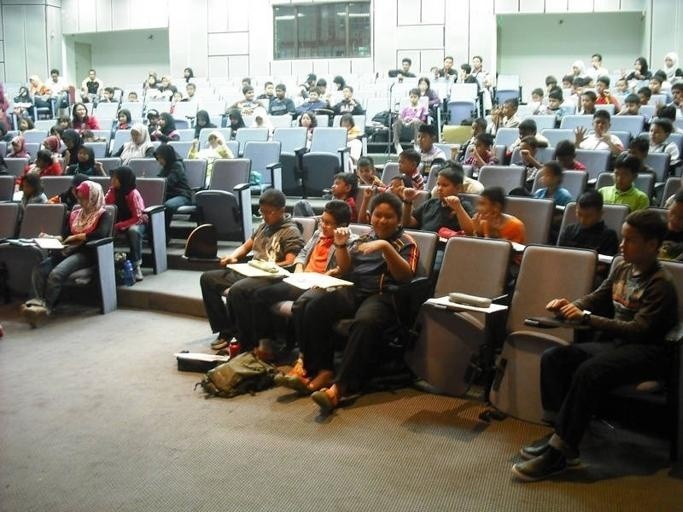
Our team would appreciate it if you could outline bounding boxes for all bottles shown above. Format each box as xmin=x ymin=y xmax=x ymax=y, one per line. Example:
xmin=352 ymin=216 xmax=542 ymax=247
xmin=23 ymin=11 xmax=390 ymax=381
xmin=123 ymin=260 xmax=135 ymax=285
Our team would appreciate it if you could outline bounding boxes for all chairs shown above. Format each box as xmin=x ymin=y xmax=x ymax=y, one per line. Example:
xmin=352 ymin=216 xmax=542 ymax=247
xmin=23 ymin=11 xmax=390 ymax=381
xmin=171 ymin=78 xmax=283 ymax=242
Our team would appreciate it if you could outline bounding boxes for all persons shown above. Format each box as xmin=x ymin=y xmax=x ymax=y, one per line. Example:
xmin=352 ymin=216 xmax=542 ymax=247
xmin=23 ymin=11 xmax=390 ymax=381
xmin=289 ymin=190 xmax=421 ymax=413
xmin=251 ymin=201 xmax=359 ymax=389
xmin=1 ymin=52 xmax=682 ymax=320
xmin=511 ymin=208 xmax=680 ymax=482
xmin=199 ymin=188 xmax=305 ymax=351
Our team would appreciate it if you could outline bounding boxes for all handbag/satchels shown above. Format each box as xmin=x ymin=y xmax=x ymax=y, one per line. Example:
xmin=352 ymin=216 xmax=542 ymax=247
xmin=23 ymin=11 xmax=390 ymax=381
xmin=200 ymin=351 xmax=284 ymax=397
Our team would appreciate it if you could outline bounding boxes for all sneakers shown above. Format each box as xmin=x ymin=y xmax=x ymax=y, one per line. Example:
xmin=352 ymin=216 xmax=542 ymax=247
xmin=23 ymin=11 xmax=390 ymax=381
xmin=511 ymin=454 xmax=566 ymax=481
xmin=211 ymin=331 xmax=230 ymax=349
xmin=20 ymin=304 xmax=48 ymax=328
xmin=521 ymin=440 xmax=580 ymax=465
xmin=134 ymin=266 xmax=144 ymax=280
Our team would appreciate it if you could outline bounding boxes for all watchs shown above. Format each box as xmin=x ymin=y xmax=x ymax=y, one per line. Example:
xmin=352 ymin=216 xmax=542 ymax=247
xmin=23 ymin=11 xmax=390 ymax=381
xmin=583 ymin=309 xmax=591 ymax=320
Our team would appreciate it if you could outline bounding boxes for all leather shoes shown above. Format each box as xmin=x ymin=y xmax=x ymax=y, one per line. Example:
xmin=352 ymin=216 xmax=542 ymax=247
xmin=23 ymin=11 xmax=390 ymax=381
xmin=274 ymin=370 xmax=337 ymax=412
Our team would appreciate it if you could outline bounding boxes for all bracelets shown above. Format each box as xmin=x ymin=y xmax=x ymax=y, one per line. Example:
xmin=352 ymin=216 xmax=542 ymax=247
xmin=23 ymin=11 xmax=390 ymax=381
xmin=334 ymin=243 xmax=347 ymax=249
